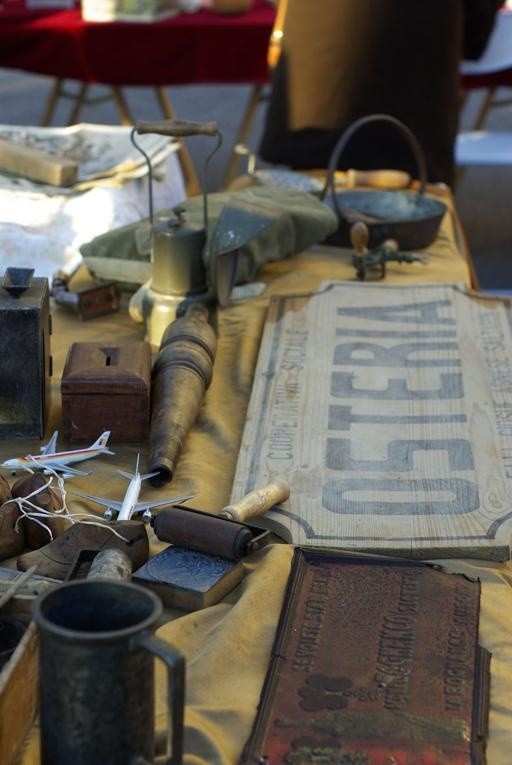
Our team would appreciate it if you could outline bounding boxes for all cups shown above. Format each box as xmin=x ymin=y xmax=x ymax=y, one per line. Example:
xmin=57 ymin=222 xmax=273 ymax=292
xmin=29 ymin=579 xmax=185 ymax=764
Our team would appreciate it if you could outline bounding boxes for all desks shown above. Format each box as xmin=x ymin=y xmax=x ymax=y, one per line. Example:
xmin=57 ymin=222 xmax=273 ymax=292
xmin=0 ymin=169 xmax=511 ymax=765
xmin=0 ymin=0 xmax=276 ymax=199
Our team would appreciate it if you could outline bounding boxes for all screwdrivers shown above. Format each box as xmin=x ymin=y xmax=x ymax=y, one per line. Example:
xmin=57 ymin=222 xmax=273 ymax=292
xmin=324 ymin=169 xmax=410 ymax=189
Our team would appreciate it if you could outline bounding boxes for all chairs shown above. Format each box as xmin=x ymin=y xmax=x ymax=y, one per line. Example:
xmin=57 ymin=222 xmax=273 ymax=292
xmin=220 ymin=2 xmax=502 ymax=195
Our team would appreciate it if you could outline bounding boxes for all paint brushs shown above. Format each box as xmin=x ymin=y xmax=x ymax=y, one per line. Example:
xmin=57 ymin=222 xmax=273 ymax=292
xmin=0 ymin=558 xmax=44 ymax=609
